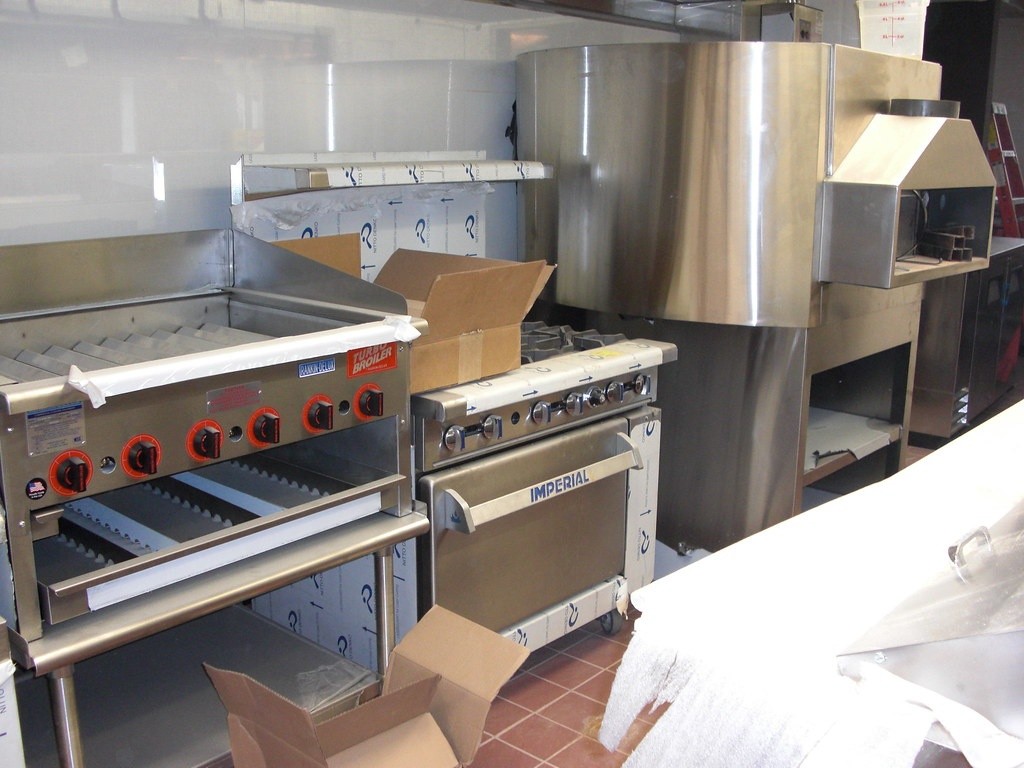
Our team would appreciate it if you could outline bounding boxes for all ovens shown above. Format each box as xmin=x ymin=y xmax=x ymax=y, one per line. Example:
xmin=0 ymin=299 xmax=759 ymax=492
xmin=417 ymin=405 xmax=661 ymax=649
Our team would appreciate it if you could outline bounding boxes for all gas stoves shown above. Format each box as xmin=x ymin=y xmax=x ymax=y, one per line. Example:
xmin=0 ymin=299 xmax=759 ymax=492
xmin=411 ymin=322 xmax=677 ymax=470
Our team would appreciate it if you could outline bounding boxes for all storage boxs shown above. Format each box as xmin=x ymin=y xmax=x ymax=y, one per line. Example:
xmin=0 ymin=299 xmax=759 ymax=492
xmin=269 ymin=233 xmax=560 ymax=395
xmin=202 ymin=601 xmax=532 ymax=768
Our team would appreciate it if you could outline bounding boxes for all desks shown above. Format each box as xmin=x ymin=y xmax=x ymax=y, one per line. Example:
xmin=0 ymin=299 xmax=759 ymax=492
xmin=600 ymin=397 xmax=1023 ymax=768
